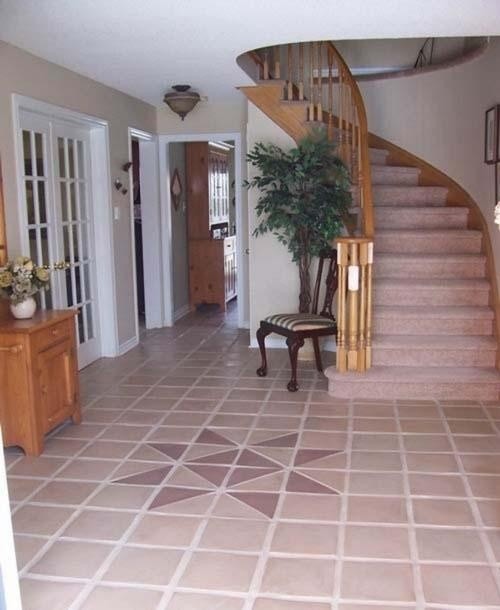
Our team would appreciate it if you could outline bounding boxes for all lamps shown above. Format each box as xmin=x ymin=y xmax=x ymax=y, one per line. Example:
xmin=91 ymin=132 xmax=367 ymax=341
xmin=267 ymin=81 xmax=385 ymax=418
xmin=164 ymin=84 xmax=200 ymax=120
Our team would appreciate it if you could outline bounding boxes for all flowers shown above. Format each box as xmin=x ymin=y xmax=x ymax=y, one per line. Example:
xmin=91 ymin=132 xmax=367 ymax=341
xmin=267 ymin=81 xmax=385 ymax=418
xmin=0 ymin=256 xmax=73 ymax=305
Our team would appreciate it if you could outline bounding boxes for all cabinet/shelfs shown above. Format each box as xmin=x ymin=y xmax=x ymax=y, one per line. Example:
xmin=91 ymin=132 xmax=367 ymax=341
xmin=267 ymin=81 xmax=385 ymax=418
xmin=186 ymin=139 xmax=239 ymax=313
xmin=0 ymin=308 xmax=80 ymax=458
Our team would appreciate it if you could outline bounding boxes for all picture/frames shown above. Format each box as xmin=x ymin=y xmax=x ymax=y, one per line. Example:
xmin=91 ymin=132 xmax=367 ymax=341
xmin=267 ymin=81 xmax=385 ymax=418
xmin=171 ymin=169 xmax=183 ymax=211
xmin=485 ymin=104 xmax=500 ymax=165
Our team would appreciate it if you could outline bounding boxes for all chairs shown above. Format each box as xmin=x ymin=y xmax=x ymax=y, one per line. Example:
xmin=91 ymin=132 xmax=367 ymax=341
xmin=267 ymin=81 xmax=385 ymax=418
xmin=256 ymin=247 xmax=351 ymax=392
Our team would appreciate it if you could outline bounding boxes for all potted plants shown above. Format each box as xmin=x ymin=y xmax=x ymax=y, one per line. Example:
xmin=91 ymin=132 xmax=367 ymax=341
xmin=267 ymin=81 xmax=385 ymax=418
xmin=239 ymin=127 xmax=354 ymax=362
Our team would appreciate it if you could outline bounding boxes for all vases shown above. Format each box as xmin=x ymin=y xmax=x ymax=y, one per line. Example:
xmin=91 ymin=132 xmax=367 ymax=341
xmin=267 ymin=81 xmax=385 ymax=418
xmin=9 ymin=297 xmax=37 ymax=320
